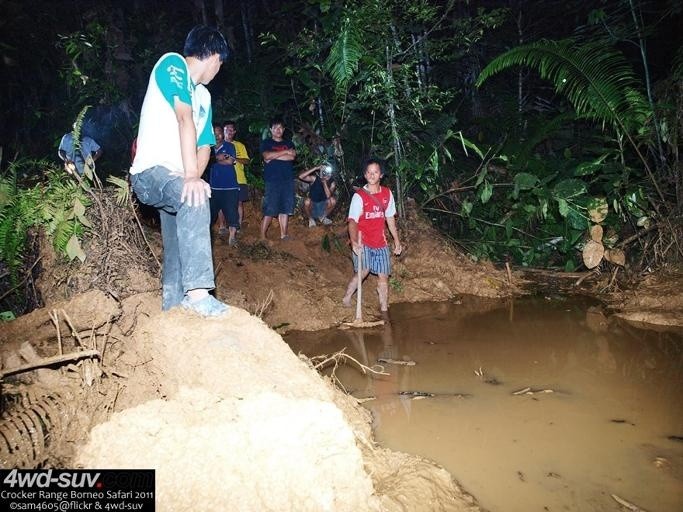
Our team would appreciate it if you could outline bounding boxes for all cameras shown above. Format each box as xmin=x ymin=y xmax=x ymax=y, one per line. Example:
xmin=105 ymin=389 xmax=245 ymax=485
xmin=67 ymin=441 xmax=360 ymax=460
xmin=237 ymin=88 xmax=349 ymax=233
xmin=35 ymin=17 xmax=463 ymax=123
xmin=320 ymin=167 xmax=332 ymax=178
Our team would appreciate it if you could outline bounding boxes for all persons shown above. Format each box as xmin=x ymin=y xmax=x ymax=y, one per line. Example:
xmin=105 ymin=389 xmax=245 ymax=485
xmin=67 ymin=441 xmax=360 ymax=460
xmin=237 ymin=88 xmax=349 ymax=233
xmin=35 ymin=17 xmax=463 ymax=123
xmin=126 ymin=26 xmax=235 ymax=318
xmin=130 ymin=125 xmax=161 ymax=226
xmin=347 ymin=310 xmax=416 ymax=450
xmin=298 ymin=160 xmax=338 ymax=230
xmin=341 ymin=160 xmax=401 ymax=311
xmin=57 ymin=116 xmax=104 ymax=190
xmin=208 ymin=124 xmax=239 ymax=250
xmin=258 ymin=119 xmax=299 ymax=242
xmin=217 ymin=121 xmax=250 ymax=236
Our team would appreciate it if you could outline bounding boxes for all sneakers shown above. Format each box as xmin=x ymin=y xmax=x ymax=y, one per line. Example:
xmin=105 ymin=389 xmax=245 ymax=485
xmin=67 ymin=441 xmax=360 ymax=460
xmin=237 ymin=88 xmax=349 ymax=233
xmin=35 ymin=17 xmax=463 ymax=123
xmin=319 ymin=217 xmax=333 ymax=225
xmin=280 ymin=234 xmax=295 ymax=241
xmin=180 ymin=293 xmax=230 ymax=318
xmin=308 ymin=218 xmax=317 ymax=228
xmin=219 ymin=229 xmax=225 ymax=235
xmin=228 ymin=236 xmax=237 ymax=247
